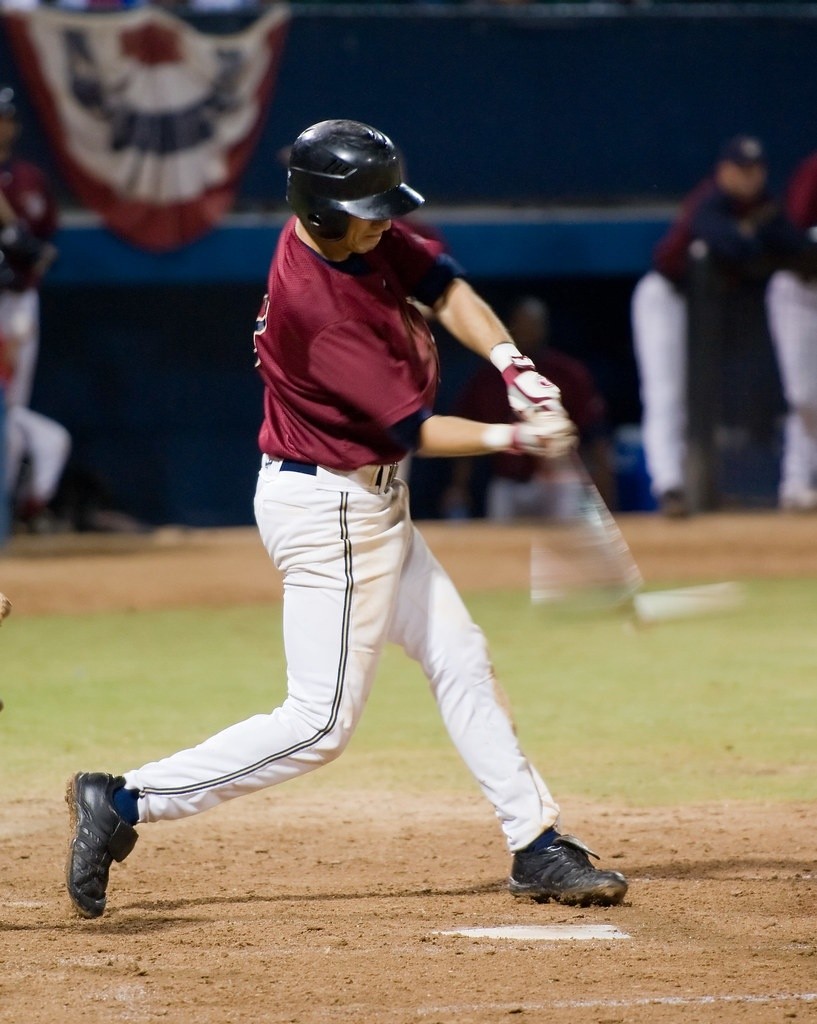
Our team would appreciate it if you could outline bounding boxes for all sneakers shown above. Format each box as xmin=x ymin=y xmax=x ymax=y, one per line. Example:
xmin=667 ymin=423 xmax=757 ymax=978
xmin=505 ymin=832 xmax=628 ymax=903
xmin=61 ymin=770 xmax=139 ymax=918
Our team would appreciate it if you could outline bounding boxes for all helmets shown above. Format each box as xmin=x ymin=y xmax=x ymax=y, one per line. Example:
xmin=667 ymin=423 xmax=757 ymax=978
xmin=284 ymin=119 xmax=426 ymax=243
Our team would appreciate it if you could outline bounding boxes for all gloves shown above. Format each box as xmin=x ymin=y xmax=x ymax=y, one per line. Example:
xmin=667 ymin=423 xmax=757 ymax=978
xmin=509 ymin=416 xmax=580 ymax=459
xmin=502 ymin=354 xmax=570 ymax=421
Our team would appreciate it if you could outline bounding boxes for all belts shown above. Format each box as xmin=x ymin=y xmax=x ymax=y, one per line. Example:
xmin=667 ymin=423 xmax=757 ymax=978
xmin=279 ymin=458 xmax=385 ymax=487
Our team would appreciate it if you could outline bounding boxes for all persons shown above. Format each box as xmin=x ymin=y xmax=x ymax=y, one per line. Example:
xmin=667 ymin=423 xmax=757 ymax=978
xmin=631 ymin=135 xmax=778 ymax=518
xmin=64 ymin=122 xmax=628 ymax=920
xmin=443 ymin=302 xmax=605 ymax=518
xmin=765 ymin=154 xmax=817 ymax=508
xmin=0 ymin=89 xmax=70 ymax=537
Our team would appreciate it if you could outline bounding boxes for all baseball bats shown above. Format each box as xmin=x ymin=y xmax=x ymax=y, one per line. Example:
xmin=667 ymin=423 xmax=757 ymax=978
xmin=532 ymin=448 xmax=646 ymax=613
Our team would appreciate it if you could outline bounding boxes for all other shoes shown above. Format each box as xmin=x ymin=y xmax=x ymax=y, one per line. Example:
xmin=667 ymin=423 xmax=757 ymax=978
xmin=654 ymin=487 xmax=690 ymax=517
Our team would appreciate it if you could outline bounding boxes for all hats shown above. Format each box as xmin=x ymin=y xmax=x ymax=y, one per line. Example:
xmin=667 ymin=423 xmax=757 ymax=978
xmin=720 ymin=133 xmax=780 ymax=171
